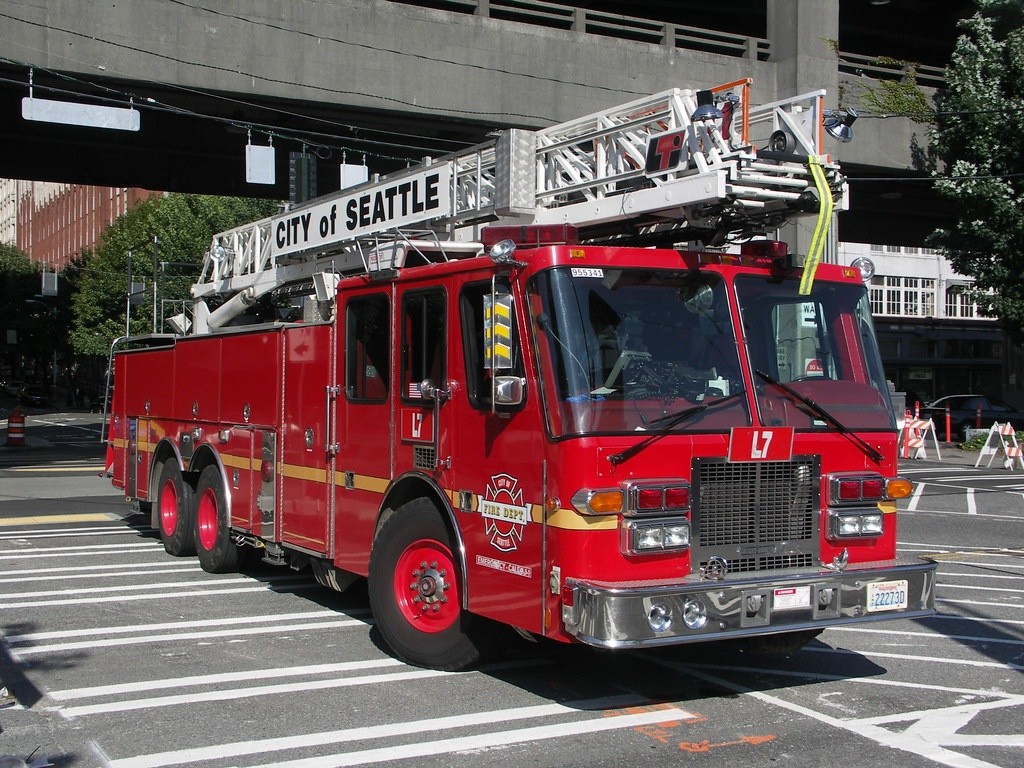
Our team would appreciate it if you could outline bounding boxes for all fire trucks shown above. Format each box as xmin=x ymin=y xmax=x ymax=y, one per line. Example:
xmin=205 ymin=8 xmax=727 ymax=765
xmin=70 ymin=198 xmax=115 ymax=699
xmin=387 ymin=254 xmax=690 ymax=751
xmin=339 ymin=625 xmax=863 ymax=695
xmin=101 ymin=79 xmax=937 ymax=672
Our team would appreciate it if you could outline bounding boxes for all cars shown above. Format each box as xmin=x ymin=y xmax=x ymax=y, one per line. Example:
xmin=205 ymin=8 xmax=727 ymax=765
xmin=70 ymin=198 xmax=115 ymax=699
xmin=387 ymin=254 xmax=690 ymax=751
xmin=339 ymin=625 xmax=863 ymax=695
xmin=90 ymin=395 xmax=112 ymax=414
xmin=911 ymin=395 xmax=1024 ymax=439
xmin=0 ymin=378 xmax=24 ymax=396
xmin=21 ymin=384 xmax=46 ymax=407
